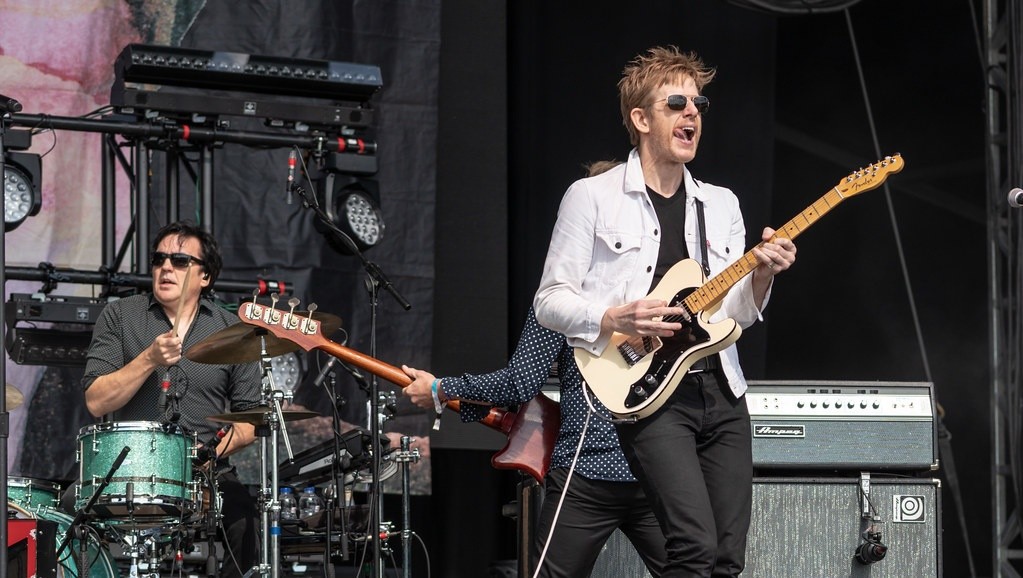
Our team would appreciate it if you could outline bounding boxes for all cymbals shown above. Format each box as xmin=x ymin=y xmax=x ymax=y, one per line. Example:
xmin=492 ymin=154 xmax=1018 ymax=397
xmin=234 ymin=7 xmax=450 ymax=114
xmin=5 ymin=382 xmax=24 ymax=411
xmin=181 ymin=308 xmax=343 ymax=365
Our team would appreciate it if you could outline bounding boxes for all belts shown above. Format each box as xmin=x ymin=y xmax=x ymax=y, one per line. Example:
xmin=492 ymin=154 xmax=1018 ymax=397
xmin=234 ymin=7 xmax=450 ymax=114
xmin=687 ymin=355 xmax=721 ymax=373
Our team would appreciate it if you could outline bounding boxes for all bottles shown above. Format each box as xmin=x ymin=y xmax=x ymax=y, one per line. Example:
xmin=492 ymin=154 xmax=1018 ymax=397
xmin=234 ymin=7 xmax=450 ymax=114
xmin=277 ymin=487 xmax=297 ymax=521
xmin=346 ymin=488 xmax=358 ymax=507
xmin=299 ymin=487 xmax=324 ymax=519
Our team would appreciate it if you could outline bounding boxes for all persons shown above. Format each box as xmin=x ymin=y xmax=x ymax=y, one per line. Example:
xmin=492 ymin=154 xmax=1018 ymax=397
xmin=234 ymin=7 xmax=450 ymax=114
xmin=529 ymin=48 xmax=799 ymax=577
xmin=82 ymin=222 xmax=265 ymax=578
xmin=401 ymin=160 xmax=673 ymax=575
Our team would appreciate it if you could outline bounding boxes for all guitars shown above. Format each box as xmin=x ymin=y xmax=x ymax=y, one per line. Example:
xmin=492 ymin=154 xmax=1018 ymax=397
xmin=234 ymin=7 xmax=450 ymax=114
xmin=572 ymin=152 xmax=905 ymax=419
xmin=238 ymin=288 xmax=561 ymax=484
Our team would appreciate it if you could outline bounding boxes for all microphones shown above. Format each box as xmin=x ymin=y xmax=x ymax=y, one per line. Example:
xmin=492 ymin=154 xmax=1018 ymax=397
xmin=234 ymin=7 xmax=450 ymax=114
xmin=856 ymin=542 xmax=887 ymax=565
xmin=313 ymin=340 xmax=347 ymax=388
xmin=192 ymin=425 xmax=232 ymax=467
xmin=158 ymin=371 xmax=170 ymax=412
xmin=286 ymin=149 xmax=297 ymax=204
xmin=1007 ymin=187 xmax=1023 ymax=208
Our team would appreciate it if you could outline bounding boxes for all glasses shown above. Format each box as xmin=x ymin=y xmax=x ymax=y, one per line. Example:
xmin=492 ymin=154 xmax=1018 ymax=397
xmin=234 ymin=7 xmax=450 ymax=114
xmin=149 ymin=251 xmax=205 ymax=268
xmin=643 ymin=95 xmax=709 ymax=113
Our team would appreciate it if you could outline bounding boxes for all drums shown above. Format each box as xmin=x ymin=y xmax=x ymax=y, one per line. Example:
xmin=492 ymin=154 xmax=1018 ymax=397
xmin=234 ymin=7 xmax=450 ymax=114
xmin=7 ymin=475 xmax=62 ymax=510
xmin=71 ymin=420 xmax=202 ymax=520
xmin=7 ymin=501 xmax=118 ymax=578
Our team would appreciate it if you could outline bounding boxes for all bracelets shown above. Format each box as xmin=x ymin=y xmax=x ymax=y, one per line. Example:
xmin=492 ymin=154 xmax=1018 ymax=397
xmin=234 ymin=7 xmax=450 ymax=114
xmin=432 ymin=377 xmax=451 ymax=431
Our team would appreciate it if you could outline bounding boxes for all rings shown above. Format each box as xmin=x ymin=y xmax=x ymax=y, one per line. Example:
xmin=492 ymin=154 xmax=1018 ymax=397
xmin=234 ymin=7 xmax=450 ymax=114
xmin=768 ymin=262 xmax=776 ymax=268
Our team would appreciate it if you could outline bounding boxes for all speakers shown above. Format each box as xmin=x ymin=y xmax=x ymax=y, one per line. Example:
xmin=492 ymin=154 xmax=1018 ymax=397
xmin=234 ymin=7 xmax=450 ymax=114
xmin=589 ymin=475 xmax=943 ymax=578
